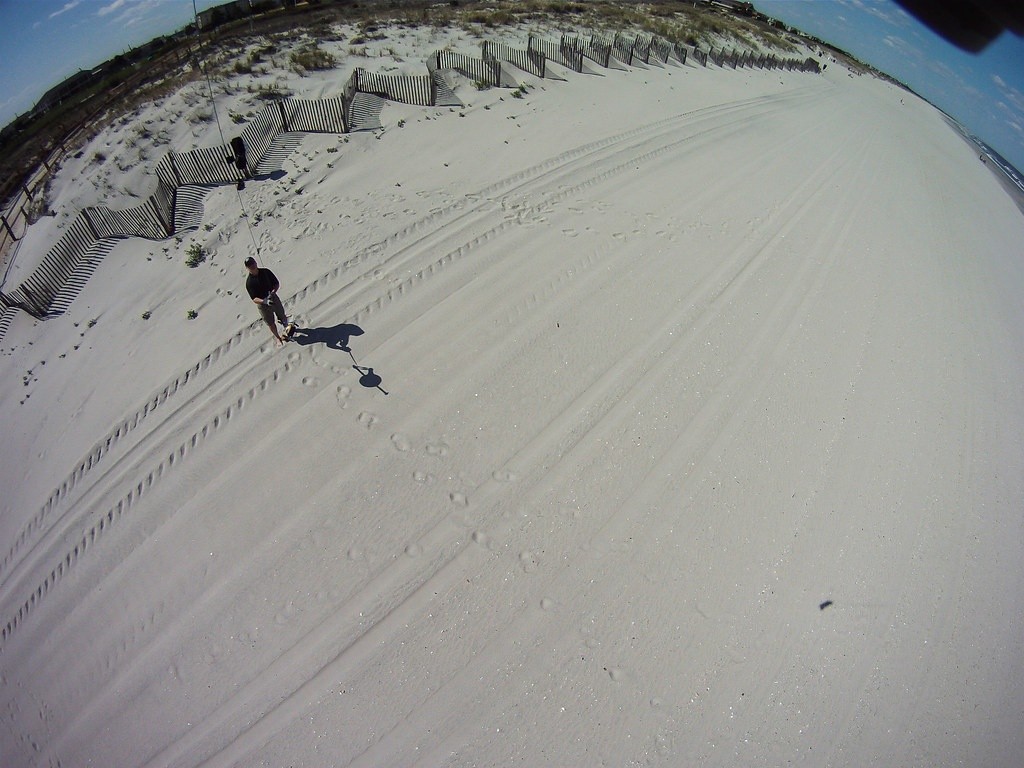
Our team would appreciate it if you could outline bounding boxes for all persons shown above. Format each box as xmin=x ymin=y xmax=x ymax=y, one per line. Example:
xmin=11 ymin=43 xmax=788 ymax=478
xmin=244 ymin=257 xmax=287 ymax=346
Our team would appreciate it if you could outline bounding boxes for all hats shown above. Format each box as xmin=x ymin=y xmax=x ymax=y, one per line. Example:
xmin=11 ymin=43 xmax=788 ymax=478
xmin=244 ymin=257 xmax=255 ymax=267
